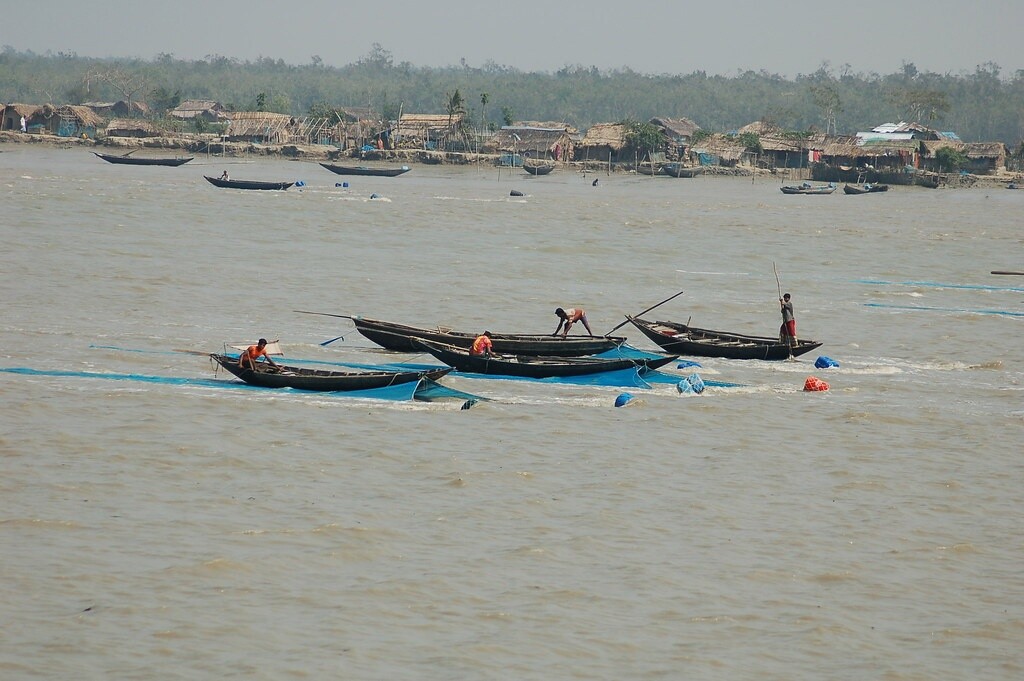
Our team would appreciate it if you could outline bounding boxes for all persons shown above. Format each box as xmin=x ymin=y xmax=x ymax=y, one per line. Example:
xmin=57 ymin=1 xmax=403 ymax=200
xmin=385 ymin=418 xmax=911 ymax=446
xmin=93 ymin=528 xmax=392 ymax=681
xmin=593 ymin=179 xmax=599 ymax=186
xmin=378 ymin=134 xmax=406 ymax=150
xmin=555 ymin=145 xmax=568 ymax=162
xmin=165 ymin=107 xmax=169 ymax=116
xmin=469 ymin=331 xmax=496 ymax=357
xmin=20 ymin=115 xmax=26 ymax=133
xmin=81 ymin=133 xmax=86 ymax=145
xmin=666 ymin=147 xmax=679 ymax=161
xmin=238 ymin=339 xmax=284 ymax=372
xmin=780 ymin=293 xmax=798 ymax=345
xmin=220 ymin=170 xmax=229 ymax=181
xmin=552 ymin=308 xmax=592 ymax=338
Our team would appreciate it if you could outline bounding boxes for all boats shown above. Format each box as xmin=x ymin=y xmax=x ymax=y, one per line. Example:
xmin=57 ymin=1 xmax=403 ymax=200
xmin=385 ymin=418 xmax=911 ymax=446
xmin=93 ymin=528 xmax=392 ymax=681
xmin=842 ymin=172 xmax=889 ymax=194
xmin=318 ymin=163 xmax=412 ymax=177
xmin=522 ymin=164 xmax=556 ymax=175
xmin=356 ymin=325 xmax=680 ymax=378
xmin=204 ymin=175 xmax=294 ymax=191
xmin=420 ymin=151 xmax=444 ymax=165
xmin=92 ymin=149 xmax=194 ymax=167
xmin=637 ymin=160 xmax=704 ymax=178
xmin=293 ymin=311 xmax=628 ymax=352
xmin=172 ymin=342 xmax=458 ymax=392
xmin=625 ymin=314 xmax=824 ymax=362
xmin=911 ymin=164 xmax=942 ymax=189
xmin=779 ymin=181 xmax=838 ymax=194
